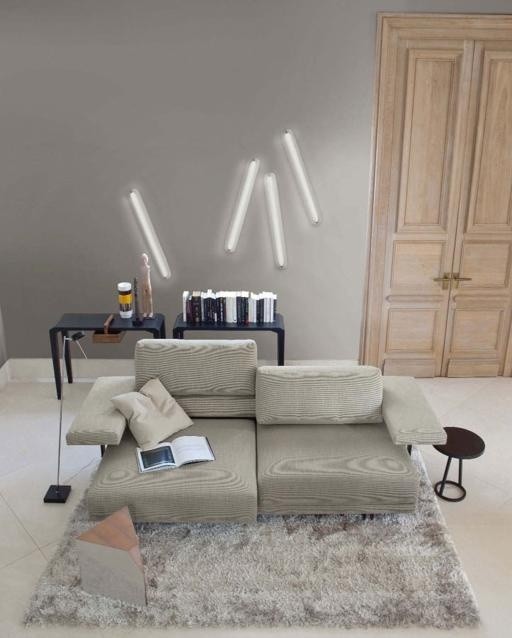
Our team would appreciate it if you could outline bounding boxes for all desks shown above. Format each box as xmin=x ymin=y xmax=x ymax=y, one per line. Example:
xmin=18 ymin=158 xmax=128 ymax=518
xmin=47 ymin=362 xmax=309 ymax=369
xmin=49 ymin=313 xmax=285 ymax=400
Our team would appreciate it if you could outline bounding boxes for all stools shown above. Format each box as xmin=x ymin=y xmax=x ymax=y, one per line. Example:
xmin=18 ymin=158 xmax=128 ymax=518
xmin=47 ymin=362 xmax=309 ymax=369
xmin=433 ymin=426 xmax=485 ymax=503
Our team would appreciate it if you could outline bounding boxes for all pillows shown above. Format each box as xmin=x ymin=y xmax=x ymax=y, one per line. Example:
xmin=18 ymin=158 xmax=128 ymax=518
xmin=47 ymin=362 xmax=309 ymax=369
xmin=111 ymin=377 xmax=194 ymax=453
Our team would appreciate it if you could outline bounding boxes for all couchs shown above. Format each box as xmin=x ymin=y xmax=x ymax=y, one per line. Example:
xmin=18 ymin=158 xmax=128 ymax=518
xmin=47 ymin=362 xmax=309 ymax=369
xmin=64 ymin=338 xmax=448 ymax=527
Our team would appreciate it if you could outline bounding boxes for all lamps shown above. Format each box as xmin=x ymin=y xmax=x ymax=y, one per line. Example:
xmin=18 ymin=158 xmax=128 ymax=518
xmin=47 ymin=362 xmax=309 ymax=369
xmin=43 ymin=331 xmax=86 ymax=503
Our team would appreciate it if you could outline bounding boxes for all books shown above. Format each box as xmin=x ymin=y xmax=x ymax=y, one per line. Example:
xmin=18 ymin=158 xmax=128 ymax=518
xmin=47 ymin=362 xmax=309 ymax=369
xmin=134 ymin=433 xmax=215 ymax=474
xmin=182 ymin=289 xmax=278 ymax=325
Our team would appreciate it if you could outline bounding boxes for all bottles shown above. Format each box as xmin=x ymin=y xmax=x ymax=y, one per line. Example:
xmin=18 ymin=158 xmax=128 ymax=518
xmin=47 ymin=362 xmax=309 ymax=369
xmin=117 ymin=281 xmax=133 ymax=319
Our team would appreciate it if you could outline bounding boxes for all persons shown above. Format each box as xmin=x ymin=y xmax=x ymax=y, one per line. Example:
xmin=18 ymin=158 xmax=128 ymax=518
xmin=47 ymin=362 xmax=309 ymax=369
xmin=139 ymin=253 xmax=153 ymax=318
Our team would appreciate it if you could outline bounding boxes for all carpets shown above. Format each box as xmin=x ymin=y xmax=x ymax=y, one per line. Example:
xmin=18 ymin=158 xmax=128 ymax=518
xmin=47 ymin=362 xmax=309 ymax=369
xmin=20 ymin=444 xmax=483 ymax=630
